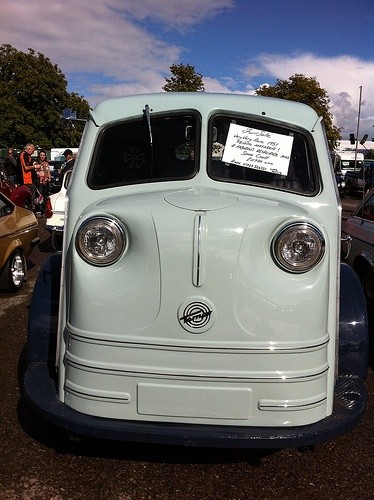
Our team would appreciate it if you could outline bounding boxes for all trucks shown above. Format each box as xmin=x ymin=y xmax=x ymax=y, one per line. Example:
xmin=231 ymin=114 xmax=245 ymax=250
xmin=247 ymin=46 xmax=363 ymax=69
xmin=333 ymin=151 xmax=365 ymax=187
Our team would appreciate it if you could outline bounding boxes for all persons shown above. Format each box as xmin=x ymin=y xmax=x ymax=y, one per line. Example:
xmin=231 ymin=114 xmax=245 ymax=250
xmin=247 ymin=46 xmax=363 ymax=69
xmin=59 ymin=149 xmax=75 ymax=187
xmin=16 ymin=144 xmax=41 ymax=206
xmin=33 ymin=151 xmax=51 ymax=215
xmin=3 ymin=148 xmax=17 ymax=183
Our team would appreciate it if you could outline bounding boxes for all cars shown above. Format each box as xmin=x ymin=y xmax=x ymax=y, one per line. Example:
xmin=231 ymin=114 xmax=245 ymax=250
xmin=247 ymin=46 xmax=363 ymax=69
xmin=15 ymin=91 xmax=371 ymax=455
xmin=0 ymin=143 xmax=79 ymax=214
xmin=44 ymin=169 xmax=73 ymax=232
xmin=341 ymin=187 xmax=374 ymax=305
xmin=0 ymin=190 xmax=41 ymax=294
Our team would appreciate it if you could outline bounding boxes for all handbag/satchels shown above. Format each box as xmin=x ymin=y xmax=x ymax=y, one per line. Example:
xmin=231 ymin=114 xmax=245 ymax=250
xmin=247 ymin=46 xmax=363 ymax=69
xmin=33 ymin=188 xmax=44 ymax=205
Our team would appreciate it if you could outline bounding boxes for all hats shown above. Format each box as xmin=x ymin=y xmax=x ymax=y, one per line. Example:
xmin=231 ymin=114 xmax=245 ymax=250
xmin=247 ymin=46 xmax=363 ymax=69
xmin=62 ymin=149 xmax=72 ymax=155
